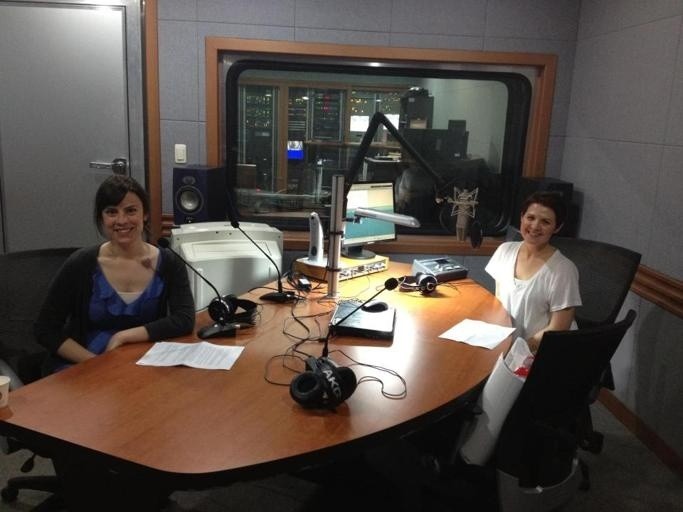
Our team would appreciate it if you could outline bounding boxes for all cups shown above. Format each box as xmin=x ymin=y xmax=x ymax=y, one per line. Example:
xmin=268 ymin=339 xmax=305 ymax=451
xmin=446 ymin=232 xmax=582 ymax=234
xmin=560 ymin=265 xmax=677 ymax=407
xmin=0 ymin=375 xmax=11 ymax=409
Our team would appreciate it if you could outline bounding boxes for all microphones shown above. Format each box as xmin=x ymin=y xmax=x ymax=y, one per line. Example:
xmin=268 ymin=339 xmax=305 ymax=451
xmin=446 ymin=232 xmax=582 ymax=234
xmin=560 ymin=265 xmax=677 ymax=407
xmin=452 ymin=187 xmax=477 ymax=241
xmin=322 ymin=278 xmax=398 ymax=357
xmin=157 ymin=237 xmax=236 ymax=339
xmin=231 ymin=219 xmax=295 ymax=302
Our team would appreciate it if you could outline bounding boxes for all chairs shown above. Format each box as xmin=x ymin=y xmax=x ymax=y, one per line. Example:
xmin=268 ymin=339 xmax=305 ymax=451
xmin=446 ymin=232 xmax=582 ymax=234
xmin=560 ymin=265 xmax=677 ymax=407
xmin=475 ymin=236 xmax=640 ymax=466
xmin=0 ymin=240 xmax=98 ymax=509
xmin=420 ymin=303 xmax=636 ymax=507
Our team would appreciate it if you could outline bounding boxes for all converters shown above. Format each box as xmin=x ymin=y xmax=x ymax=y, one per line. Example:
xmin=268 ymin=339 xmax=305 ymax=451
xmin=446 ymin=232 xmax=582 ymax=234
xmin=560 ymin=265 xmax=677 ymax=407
xmin=298 ymin=278 xmax=312 ymax=289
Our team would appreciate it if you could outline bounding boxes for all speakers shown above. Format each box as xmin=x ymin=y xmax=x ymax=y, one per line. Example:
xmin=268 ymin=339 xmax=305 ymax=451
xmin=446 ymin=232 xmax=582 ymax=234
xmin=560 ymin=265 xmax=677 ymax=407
xmin=172 ymin=164 xmax=229 ymax=224
xmin=308 ymin=211 xmax=324 ymax=261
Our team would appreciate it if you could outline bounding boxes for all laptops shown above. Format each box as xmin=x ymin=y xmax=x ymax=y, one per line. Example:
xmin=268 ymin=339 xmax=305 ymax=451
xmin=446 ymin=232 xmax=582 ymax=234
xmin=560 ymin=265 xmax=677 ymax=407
xmin=329 ymin=298 xmax=395 ymax=341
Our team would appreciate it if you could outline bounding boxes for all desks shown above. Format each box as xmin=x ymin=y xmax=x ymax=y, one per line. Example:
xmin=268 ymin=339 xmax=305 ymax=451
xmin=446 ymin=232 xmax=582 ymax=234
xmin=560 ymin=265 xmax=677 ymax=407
xmin=1 ymin=255 xmax=515 ymax=502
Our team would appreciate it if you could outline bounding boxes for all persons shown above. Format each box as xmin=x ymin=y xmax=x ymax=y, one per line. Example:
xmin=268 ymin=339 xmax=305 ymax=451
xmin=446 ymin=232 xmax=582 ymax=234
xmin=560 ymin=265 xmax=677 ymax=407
xmin=484 ymin=190 xmax=584 ymax=365
xmin=35 ymin=174 xmax=195 ymax=374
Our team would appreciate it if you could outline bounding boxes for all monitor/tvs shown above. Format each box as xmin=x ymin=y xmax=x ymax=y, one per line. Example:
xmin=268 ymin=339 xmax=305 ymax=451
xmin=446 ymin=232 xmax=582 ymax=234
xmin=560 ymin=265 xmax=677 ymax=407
xmin=287 ymin=140 xmax=304 ymax=161
xmin=341 ymin=180 xmax=397 ymax=259
xmin=383 ymin=113 xmax=400 ymax=130
xmin=350 ymin=114 xmax=369 ymax=132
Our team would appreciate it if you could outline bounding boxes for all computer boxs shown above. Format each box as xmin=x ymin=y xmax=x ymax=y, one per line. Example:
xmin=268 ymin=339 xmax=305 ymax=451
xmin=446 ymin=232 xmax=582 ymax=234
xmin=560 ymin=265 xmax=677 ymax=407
xmin=295 ymin=250 xmax=389 ymax=284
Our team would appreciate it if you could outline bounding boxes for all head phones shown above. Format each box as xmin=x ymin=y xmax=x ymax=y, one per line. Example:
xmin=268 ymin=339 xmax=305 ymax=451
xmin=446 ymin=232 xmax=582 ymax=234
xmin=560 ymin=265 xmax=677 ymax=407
xmin=397 ymin=272 xmax=437 ymax=294
xmin=291 ymin=356 xmax=356 ymax=408
xmin=208 ymin=294 xmax=256 ymax=324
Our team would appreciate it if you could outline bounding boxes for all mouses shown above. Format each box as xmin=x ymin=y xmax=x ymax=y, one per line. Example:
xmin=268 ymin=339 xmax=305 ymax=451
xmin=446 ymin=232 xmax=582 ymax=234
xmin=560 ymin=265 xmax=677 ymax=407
xmin=363 ymin=300 xmax=388 ymax=312
